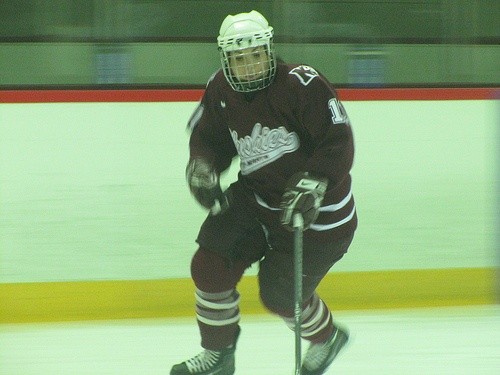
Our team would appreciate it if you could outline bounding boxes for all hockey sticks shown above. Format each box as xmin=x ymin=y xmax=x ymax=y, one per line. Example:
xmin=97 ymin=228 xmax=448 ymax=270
xmin=292 ymin=212 xmax=304 ymax=375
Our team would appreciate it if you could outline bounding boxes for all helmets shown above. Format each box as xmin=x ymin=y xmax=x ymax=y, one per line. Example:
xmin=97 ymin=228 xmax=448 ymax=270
xmin=217 ymin=10 xmax=277 ymax=92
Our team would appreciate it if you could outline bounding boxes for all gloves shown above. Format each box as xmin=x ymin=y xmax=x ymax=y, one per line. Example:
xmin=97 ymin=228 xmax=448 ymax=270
xmin=185 ymin=160 xmax=225 ymax=210
xmin=279 ymin=172 xmax=329 ymax=232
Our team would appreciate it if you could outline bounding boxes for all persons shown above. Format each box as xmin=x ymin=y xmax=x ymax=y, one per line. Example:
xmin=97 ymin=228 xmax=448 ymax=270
xmin=171 ymin=10 xmax=359 ymax=375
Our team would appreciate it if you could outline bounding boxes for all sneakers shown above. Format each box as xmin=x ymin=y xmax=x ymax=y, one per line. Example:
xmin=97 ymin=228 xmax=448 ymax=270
xmin=301 ymin=326 xmax=348 ymax=375
xmin=170 ymin=348 xmax=235 ymax=375
